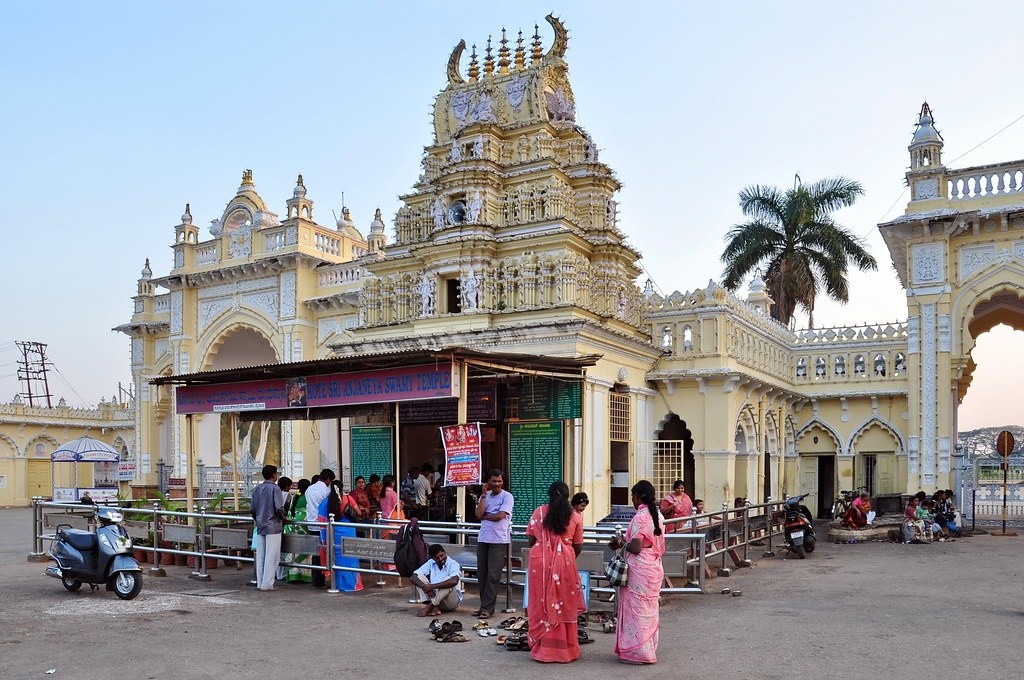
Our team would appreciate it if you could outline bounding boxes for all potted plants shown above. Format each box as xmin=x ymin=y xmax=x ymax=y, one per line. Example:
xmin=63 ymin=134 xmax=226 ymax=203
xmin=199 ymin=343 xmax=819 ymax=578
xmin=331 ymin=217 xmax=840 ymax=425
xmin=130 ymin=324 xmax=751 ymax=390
xmin=114 ymin=491 xmax=249 ymax=569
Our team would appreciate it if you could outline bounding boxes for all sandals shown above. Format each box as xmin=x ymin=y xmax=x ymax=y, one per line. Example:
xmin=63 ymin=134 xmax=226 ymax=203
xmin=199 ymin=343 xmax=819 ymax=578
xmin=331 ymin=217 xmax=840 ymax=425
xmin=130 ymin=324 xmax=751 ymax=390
xmin=472 ymin=610 xmax=482 ymax=616
xmin=478 ymin=611 xmax=491 ymax=619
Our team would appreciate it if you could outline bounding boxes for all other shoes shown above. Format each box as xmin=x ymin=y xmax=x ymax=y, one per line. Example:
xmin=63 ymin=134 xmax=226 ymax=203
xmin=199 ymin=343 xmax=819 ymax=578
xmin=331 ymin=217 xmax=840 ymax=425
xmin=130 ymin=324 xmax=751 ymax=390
xmin=832 ymin=529 xmax=974 ymax=545
xmin=262 ymin=587 xmax=279 ymax=592
xmin=608 ymin=619 xmax=616 ymax=631
xmin=472 ymin=623 xmax=485 ymax=630
xmin=603 ymin=622 xmax=610 ymax=632
xmin=312 ymin=584 xmax=330 ymax=589
xmin=487 ymin=628 xmax=497 ymax=636
xmin=619 ymin=659 xmax=645 ymax=665
xmin=291 ymin=580 xmax=300 ymax=584
xmin=428 ymin=618 xmax=470 ymax=642
xmin=478 ymin=619 xmax=489 ymax=628
xmin=278 ymin=577 xmax=286 ymax=581
xmin=477 ymin=629 xmax=488 ymax=637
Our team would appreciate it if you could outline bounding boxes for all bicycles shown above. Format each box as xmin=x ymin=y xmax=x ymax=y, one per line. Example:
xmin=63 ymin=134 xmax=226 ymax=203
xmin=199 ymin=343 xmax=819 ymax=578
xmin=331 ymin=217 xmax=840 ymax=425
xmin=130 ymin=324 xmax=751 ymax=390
xmin=831 ymin=485 xmax=869 ymax=529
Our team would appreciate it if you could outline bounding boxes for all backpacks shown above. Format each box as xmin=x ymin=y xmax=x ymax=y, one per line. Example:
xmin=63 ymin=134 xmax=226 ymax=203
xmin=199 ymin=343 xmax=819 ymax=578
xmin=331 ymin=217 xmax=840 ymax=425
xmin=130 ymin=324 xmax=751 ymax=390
xmin=367 ymin=485 xmax=382 ymax=514
xmin=394 ymin=523 xmax=420 ymax=577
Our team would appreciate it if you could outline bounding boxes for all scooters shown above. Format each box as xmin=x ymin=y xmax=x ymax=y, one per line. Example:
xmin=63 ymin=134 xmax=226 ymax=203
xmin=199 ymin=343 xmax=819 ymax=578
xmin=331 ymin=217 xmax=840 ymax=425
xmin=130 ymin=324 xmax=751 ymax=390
xmin=778 ymin=492 xmax=816 ymax=559
xmin=45 ymin=495 xmax=145 ymax=601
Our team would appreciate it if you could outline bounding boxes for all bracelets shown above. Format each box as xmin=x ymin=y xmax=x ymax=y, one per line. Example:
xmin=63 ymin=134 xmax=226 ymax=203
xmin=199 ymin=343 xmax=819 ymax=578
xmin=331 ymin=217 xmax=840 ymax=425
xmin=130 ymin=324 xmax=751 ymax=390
xmin=481 ymin=495 xmax=486 ymax=499
xmin=617 ymin=538 xmax=626 ymax=547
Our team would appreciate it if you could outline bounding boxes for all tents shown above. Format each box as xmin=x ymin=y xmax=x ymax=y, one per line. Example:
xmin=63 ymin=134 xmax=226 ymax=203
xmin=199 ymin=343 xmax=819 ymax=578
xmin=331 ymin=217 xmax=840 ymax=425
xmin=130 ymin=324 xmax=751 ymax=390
xmin=51 ymin=434 xmax=120 ymax=489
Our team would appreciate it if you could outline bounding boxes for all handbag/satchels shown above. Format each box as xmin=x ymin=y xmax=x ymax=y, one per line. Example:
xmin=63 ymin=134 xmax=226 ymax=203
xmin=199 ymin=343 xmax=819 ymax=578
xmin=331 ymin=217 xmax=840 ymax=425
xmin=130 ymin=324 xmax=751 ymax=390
xmin=945 ymin=506 xmax=954 ymax=521
xmin=951 ymin=508 xmax=961 ymax=527
xmin=604 ymin=543 xmax=629 ymax=587
xmin=866 ymin=510 xmax=876 ymax=524
xmin=344 ymin=495 xmax=359 ymax=523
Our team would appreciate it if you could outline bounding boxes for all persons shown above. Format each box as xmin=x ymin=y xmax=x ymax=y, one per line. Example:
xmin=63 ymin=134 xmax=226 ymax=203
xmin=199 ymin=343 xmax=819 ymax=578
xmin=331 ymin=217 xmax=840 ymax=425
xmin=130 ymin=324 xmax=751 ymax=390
xmin=250 ymin=465 xmax=290 ymax=591
xmin=410 ymin=544 xmax=464 ymax=617
xmin=276 ymin=463 xmax=488 ymax=589
xmin=846 ymin=493 xmax=872 ymax=529
xmin=905 ymin=489 xmax=961 ymax=544
xmin=607 ymin=480 xmax=665 ymax=664
xmin=526 ymin=481 xmax=589 ymax=662
xmin=660 ymin=480 xmax=723 ymax=552
xmin=471 ymin=469 xmax=514 ymax=619
xmin=420 ymin=277 xmax=430 ymax=316
xmin=461 ymin=276 xmax=482 ymax=308
xmin=159 ymin=517 xmax=177 ymax=530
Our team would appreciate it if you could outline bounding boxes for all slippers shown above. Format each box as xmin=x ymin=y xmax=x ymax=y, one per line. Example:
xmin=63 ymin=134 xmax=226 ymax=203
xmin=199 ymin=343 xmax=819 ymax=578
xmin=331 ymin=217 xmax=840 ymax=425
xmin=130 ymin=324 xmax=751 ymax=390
xmin=496 ymin=616 xmax=595 ymax=651
